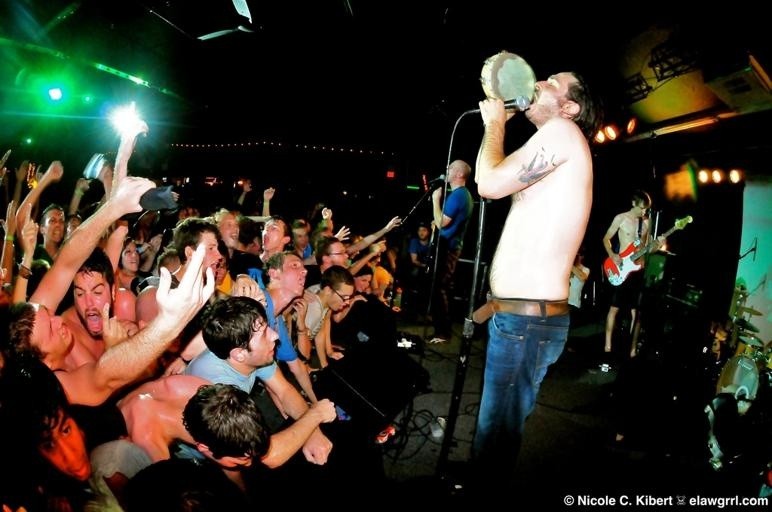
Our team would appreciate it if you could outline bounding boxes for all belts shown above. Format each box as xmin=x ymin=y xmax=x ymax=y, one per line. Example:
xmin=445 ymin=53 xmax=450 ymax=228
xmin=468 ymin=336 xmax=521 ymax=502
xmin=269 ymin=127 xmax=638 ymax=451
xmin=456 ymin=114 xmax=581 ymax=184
xmin=472 ymin=297 xmax=568 ymax=324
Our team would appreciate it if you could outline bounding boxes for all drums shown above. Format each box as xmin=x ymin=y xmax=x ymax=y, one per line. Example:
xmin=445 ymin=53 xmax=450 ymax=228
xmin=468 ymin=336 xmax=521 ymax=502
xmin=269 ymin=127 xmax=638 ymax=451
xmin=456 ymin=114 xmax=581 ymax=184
xmin=764 ymin=346 xmax=772 ymax=370
xmin=480 ymin=50 xmax=536 ymax=113
xmin=716 ymin=352 xmax=772 ymax=417
xmin=604 ymin=215 xmax=693 ymax=287
xmin=733 ymin=335 xmax=764 ymax=356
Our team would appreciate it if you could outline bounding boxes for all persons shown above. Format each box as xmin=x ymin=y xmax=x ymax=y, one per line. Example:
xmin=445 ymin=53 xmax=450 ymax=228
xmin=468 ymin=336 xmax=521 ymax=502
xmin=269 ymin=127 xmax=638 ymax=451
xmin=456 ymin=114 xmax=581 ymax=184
xmin=603 ymin=190 xmax=668 ymax=359
xmin=1 ymin=92 xmax=476 ymax=512
xmin=568 ymin=247 xmax=592 ymax=326
xmin=474 ymin=70 xmax=595 ymax=470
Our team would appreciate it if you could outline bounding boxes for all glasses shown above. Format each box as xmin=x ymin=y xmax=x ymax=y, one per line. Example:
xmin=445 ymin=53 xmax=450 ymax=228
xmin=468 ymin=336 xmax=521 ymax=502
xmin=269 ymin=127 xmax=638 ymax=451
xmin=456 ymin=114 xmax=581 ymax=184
xmin=330 ymin=251 xmax=348 ymax=256
xmin=332 ymin=288 xmax=353 ymax=302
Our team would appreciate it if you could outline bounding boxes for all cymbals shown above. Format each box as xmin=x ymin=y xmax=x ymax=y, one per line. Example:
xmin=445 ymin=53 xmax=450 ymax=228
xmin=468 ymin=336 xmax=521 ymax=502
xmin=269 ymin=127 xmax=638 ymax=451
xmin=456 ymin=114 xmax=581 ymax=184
xmin=735 ymin=318 xmax=760 ymax=333
xmin=741 ymin=306 xmax=762 ymax=317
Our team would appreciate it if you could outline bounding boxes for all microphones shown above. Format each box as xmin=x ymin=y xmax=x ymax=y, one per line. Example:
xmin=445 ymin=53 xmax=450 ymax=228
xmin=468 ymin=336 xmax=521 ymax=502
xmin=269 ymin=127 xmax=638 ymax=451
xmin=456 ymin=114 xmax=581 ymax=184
xmin=472 ymin=95 xmax=530 ymax=113
xmin=426 ymin=174 xmax=446 ymax=185
xmin=753 ymin=240 xmax=757 ymax=260
